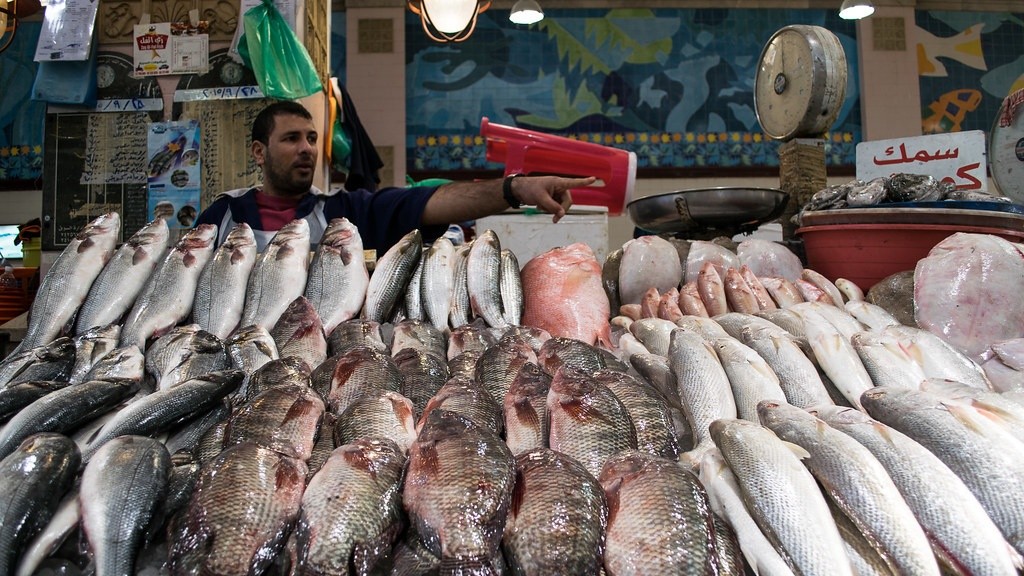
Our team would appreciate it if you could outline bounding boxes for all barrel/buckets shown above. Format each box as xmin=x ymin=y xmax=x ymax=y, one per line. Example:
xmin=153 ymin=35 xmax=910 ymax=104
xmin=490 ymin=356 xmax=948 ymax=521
xmin=21 ymin=238 xmax=40 ymax=267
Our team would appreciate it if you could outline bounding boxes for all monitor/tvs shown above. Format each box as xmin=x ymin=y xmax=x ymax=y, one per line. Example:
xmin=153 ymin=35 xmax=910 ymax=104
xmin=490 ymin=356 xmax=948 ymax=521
xmin=0 ymin=224 xmax=23 ymax=259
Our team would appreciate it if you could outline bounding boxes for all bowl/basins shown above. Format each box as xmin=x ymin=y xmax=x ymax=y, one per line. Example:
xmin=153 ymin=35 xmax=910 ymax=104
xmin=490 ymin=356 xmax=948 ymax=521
xmin=796 ymin=202 xmax=1024 ymax=296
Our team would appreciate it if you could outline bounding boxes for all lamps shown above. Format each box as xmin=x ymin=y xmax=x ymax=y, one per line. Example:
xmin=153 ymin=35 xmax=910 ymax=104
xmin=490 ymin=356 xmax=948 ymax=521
xmin=838 ymin=0 xmax=875 ymax=20
xmin=0 ymin=0 xmax=17 ymax=51
xmin=407 ymin=0 xmax=492 ymax=41
xmin=509 ymin=0 xmax=544 ymax=25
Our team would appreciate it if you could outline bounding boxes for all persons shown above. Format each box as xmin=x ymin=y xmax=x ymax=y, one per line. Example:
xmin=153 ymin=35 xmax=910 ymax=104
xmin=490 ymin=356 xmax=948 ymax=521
xmin=194 ymin=100 xmax=596 ymax=253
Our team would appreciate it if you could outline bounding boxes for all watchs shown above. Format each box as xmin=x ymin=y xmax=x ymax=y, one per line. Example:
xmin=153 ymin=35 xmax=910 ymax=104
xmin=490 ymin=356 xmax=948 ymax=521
xmin=504 ymin=173 xmax=525 ymax=209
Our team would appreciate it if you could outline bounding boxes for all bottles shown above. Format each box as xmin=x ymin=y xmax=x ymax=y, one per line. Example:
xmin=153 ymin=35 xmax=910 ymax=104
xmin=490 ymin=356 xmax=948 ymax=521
xmin=0 ymin=265 xmax=18 ymax=288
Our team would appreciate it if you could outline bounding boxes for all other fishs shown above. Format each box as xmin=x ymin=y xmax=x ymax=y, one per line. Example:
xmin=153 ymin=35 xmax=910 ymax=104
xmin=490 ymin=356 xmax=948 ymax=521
xmin=0 ymin=203 xmax=1019 ymax=576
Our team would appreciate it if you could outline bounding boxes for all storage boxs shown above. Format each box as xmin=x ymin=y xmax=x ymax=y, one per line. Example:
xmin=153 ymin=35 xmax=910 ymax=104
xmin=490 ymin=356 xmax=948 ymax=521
xmin=477 ymin=206 xmax=610 ymax=273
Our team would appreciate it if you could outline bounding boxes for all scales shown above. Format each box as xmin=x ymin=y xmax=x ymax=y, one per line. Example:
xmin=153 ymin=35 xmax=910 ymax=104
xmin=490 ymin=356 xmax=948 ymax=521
xmin=624 ymin=24 xmax=849 ymax=272
xmin=986 ymin=87 xmax=1024 ymax=205
